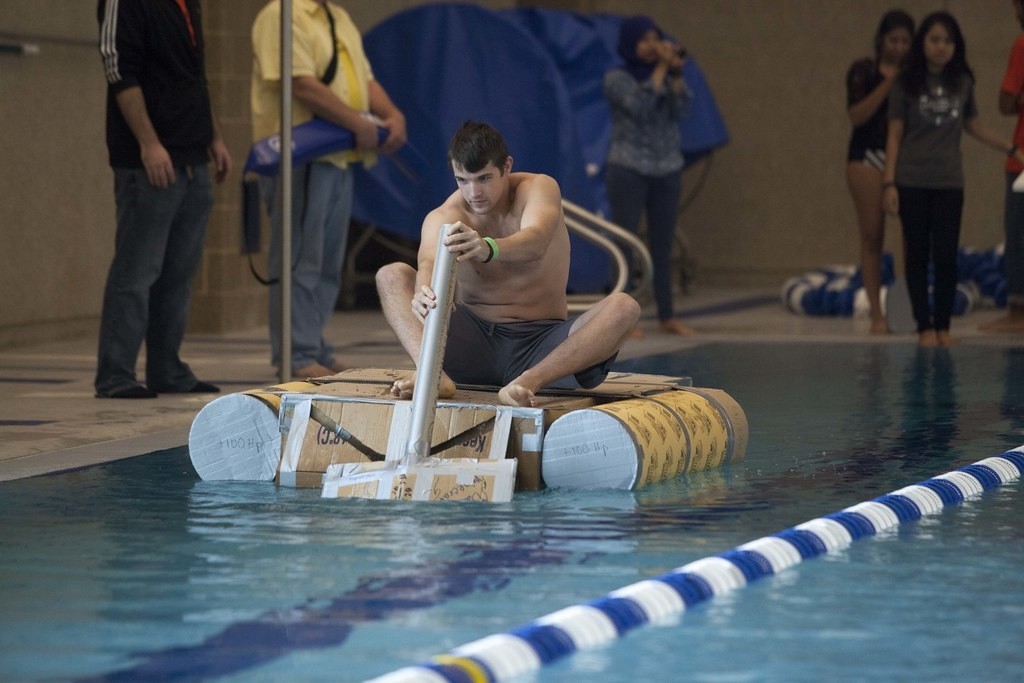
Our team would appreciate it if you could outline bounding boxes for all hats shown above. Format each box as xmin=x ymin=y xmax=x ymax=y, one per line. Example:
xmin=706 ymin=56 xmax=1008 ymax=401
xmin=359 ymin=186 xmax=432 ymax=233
xmin=617 ymin=16 xmax=663 ymax=56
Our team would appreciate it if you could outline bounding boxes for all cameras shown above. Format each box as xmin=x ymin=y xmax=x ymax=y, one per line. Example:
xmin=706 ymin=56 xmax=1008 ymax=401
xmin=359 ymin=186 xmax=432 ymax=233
xmin=671 ymin=44 xmax=688 ymax=58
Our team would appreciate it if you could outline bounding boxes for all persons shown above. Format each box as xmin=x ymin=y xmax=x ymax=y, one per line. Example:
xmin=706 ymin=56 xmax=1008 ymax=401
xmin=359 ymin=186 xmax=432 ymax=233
xmin=977 ymin=0 xmax=1024 ymax=330
xmin=95 ymin=0 xmax=231 ymax=400
xmin=243 ymin=0 xmax=407 ymax=378
xmin=884 ymin=11 xmax=1024 ymax=346
xmin=844 ymin=9 xmax=916 ymax=335
xmin=602 ymin=14 xmax=696 ymax=340
xmin=375 ymin=119 xmax=641 ymax=407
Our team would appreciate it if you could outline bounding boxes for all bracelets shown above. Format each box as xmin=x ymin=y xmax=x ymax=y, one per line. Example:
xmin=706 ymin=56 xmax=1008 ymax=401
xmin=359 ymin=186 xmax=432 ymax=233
xmin=482 ymin=237 xmax=499 ymax=264
xmin=1009 ymin=144 xmax=1017 ymax=155
xmin=885 ymin=181 xmax=895 ymax=187
